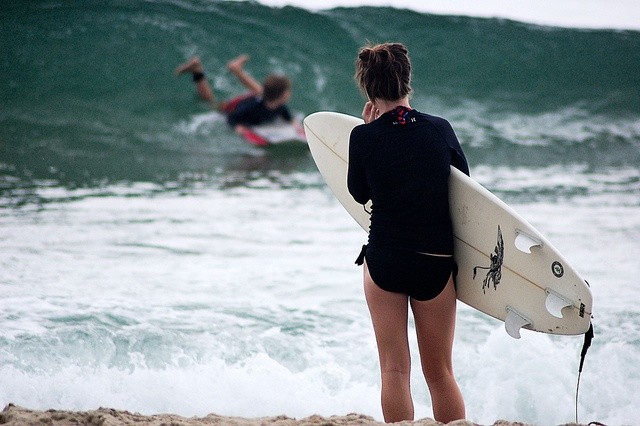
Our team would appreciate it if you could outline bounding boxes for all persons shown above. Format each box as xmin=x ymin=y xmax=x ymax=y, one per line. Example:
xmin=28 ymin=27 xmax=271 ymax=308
xmin=176 ymin=53 xmax=300 ymax=136
xmin=346 ymin=37 xmax=470 ymax=423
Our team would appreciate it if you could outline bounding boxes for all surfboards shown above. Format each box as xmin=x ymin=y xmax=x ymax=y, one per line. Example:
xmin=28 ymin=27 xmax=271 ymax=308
xmin=303 ymin=112 xmax=592 ymax=339
